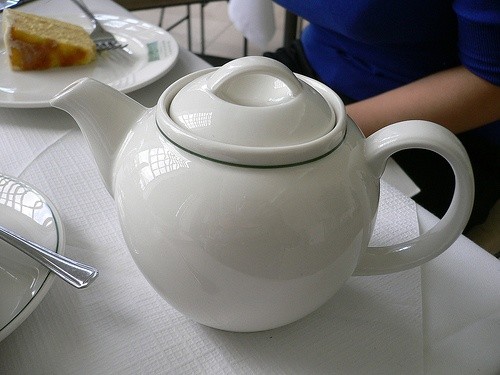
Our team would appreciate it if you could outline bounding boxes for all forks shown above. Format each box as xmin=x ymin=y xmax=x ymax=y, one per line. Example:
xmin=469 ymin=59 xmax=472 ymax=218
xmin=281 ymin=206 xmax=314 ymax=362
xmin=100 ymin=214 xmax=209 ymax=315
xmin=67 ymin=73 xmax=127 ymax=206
xmin=71 ymin=0 xmax=128 ymax=52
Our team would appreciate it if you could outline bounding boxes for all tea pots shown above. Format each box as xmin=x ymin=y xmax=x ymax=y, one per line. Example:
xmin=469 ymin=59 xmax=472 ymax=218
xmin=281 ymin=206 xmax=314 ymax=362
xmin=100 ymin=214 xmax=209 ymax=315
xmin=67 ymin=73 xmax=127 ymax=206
xmin=48 ymin=55 xmax=475 ymax=333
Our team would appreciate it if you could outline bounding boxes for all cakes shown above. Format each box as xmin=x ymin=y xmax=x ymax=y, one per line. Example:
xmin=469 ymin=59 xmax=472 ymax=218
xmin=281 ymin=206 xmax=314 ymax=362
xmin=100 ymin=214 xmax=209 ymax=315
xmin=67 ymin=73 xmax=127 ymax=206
xmin=2 ymin=8 xmax=97 ymax=70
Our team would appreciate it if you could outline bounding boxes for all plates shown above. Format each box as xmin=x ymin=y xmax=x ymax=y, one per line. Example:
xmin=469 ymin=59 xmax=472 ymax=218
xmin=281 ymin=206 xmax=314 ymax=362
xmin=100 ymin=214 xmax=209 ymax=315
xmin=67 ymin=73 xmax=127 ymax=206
xmin=0 ymin=172 xmax=66 ymax=343
xmin=0 ymin=13 xmax=180 ymax=108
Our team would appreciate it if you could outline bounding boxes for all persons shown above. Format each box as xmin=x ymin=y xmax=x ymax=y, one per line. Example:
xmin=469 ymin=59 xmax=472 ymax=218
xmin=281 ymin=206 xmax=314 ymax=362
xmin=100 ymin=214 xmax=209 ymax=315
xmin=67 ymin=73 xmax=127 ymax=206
xmin=263 ymin=0 xmax=500 ymax=238
